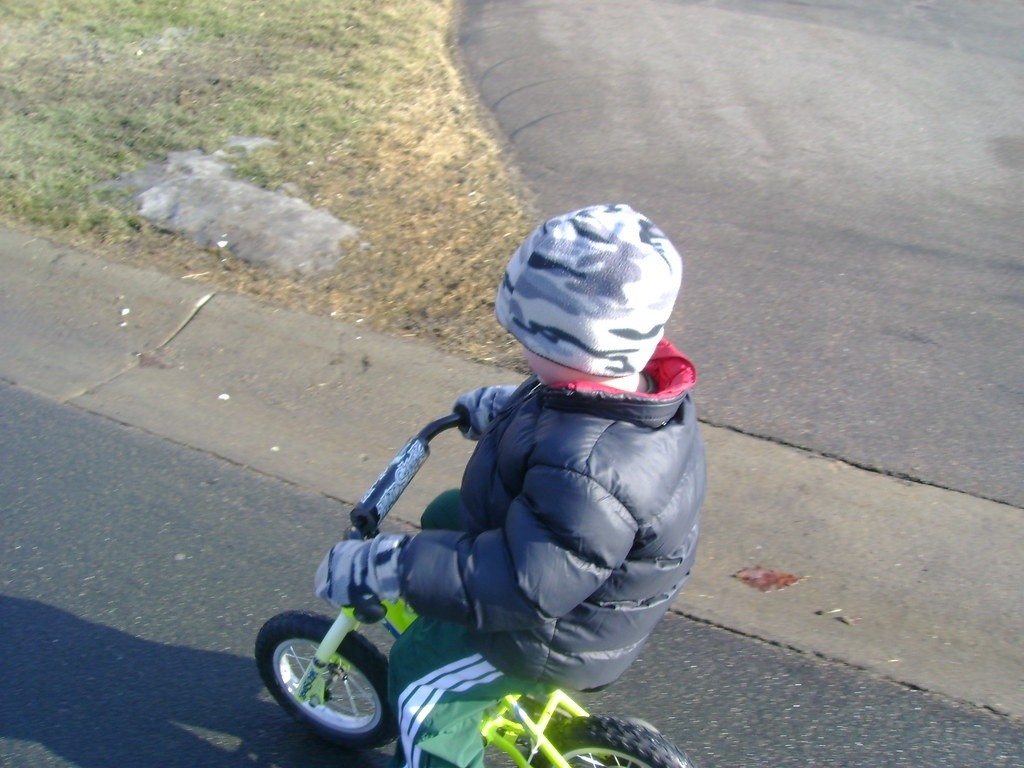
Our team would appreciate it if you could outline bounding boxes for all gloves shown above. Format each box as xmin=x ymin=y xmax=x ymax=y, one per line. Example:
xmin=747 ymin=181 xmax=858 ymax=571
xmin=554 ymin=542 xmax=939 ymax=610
xmin=452 ymin=385 xmax=518 ymax=440
xmin=315 ymin=533 xmax=409 ymax=608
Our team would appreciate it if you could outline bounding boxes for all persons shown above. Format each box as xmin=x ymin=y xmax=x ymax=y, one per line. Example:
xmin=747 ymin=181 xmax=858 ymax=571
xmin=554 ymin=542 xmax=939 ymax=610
xmin=313 ymin=201 xmax=705 ymax=768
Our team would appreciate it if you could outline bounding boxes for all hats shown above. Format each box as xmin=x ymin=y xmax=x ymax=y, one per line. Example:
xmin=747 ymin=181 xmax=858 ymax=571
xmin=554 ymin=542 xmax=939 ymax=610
xmin=496 ymin=204 xmax=683 ymax=377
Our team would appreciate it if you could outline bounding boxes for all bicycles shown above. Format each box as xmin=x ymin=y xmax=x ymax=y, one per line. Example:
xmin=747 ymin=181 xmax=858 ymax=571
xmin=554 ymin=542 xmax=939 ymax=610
xmin=254 ymin=403 xmax=698 ymax=768
